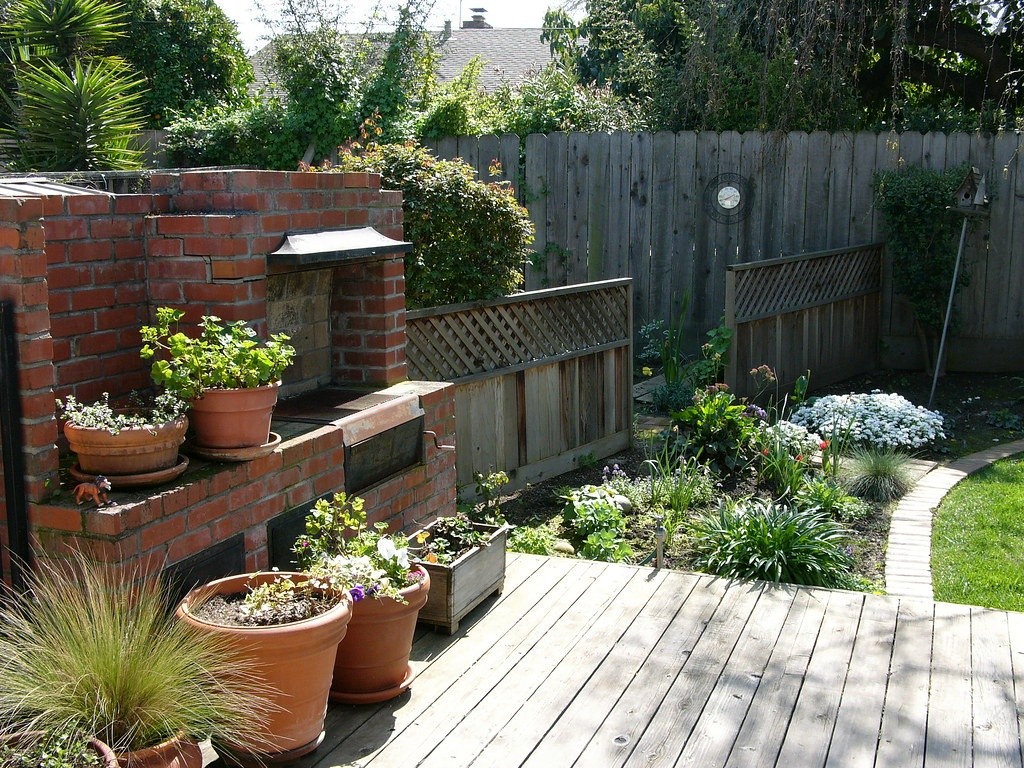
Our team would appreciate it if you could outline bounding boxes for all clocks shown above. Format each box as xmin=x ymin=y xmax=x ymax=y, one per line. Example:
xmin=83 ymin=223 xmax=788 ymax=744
xmin=717 ymin=186 xmax=740 ymax=209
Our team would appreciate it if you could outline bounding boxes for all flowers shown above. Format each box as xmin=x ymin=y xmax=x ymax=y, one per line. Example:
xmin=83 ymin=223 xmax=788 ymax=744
xmin=293 ymin=489 xmax=425 ymax=606
xmin=407 ymin=517 xmax=491 ymax=567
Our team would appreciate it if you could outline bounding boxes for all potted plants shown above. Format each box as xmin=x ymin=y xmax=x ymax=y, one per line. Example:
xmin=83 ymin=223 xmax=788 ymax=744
xmin=0 ymin=540 xmax=283 ymax=768
xmin=140 ymin=304 xmax=297 ymax=462
xmin=54 ymin=388 xmax=193 ymax=484
xmin=177 ymin=559 xmax=353 ymax=768
xmin=465 ymin=468 xmax=509 ymax=534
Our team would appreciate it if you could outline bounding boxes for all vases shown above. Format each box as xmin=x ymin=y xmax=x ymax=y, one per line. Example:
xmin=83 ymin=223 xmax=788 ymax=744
xmin=330 ymin=561 xmax=430 ymax=704
xmin=405 ymin=518 xmax=508 ymax=636
